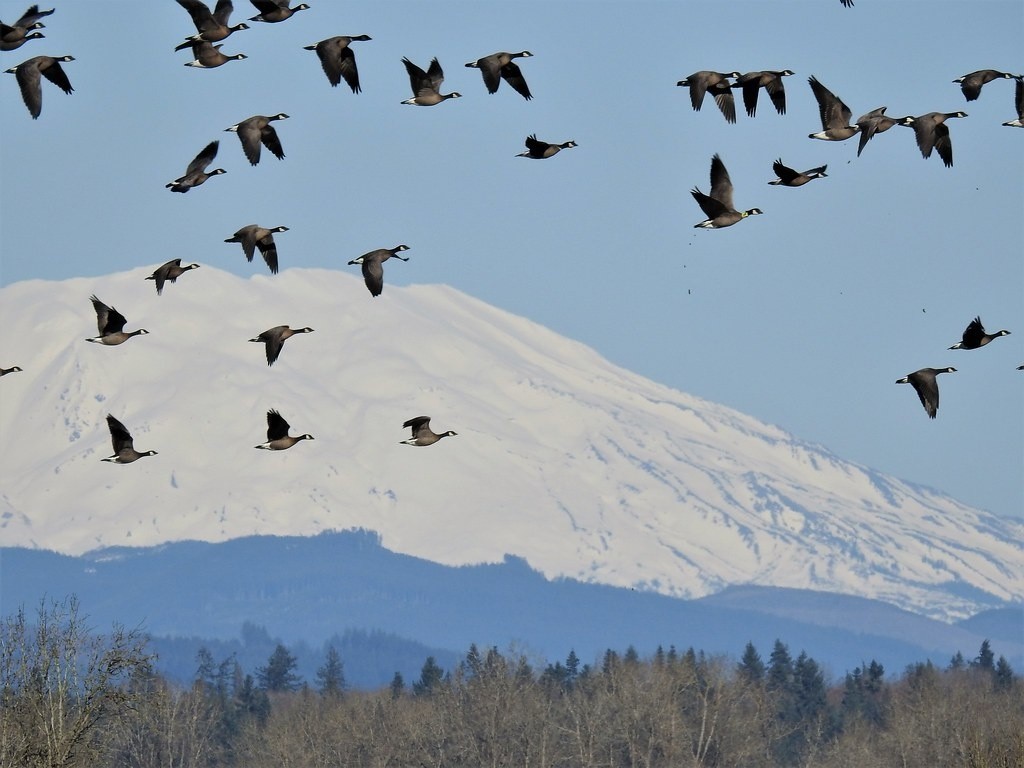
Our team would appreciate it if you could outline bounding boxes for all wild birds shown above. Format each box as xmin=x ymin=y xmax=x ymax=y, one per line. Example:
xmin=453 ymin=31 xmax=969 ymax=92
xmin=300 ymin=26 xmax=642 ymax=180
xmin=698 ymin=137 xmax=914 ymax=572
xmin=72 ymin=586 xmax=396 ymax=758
xmin=224 ymin=112 xmax=290 ymax=167
xmin=172 ymin=0 xmax=309 ymax=68
xmin=223 ymin=225 xmax=290 ymax=274
xmin=84 ymin=294 xmax=151 ymax=347
xmin=897 ymin=367 xmax=958 ymax=420
xmin=676 ymin=69 xmax=1024 ymax=229
xmin=515 ymin=133 xmax=578 ymax=160
xmin=4 ymin=55 xmax=76 ymax=119
xmin=464 ymin=51 xmax=536 ymax=100
xmin=254 ymin=408 xmax=315 ymax=450
xmin=949 ymin=317 xmax=1012 ymax=351
xmin=0 ymin=3 xmax=57 ymax=51
xmin=145 ymin=258 xmax=200 ymax=296
xmin=400 ymin=55 xmax=461 ymax=106
xmin=100 ymin=414 xmax=158 ymax=464
xmin=347 ymin=244 xmax=410 ymax=297
xmin=398 ymin=416 xmax=461 ymax=446
xmin=165 ymin=139 xmax=226 ymax=193
xmin=248 ymin=325 xmax=316 ymax=368
xmin=302 ymin=34 xmax=374 ymax=95
